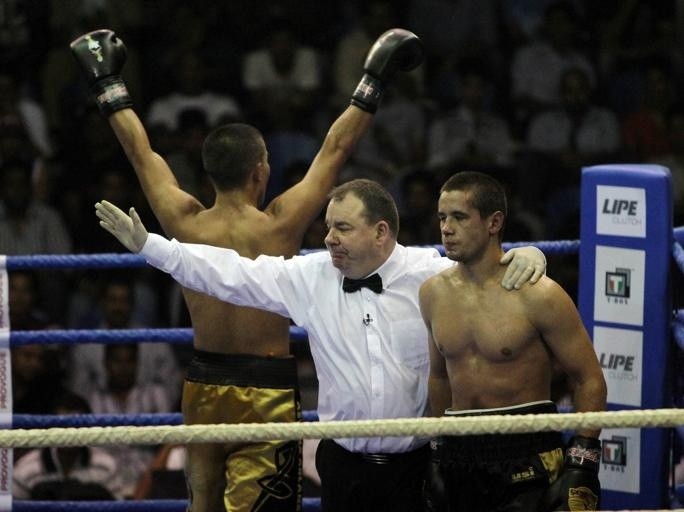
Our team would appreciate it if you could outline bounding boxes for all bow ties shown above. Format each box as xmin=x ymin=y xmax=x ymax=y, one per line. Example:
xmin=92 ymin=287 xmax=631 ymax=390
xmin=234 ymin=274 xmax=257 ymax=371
xmin=343 ymin=273 xmax=383 ymax=293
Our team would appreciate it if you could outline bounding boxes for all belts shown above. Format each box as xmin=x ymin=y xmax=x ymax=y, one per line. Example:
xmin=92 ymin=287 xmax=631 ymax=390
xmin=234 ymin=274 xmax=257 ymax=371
xmin=363 ymin=452 xmax=401 ymax=466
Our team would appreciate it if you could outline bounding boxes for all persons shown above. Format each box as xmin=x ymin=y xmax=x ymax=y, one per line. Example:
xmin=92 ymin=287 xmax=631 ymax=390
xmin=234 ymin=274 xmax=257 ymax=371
xmin=1 ymin=0 xmax=683 ymax=512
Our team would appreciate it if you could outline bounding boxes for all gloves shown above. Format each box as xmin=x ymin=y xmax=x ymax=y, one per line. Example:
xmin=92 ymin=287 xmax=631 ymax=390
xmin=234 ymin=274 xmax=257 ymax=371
xmin=352 ymin=29 xmax=420 ymax=113
xmin=423 ymin=441 xmax=453 ymax=512
xmin=548 ymin=433 xmax=602 ymax=510
xmin=71 ymin=29 xmax=133 ymax=117
